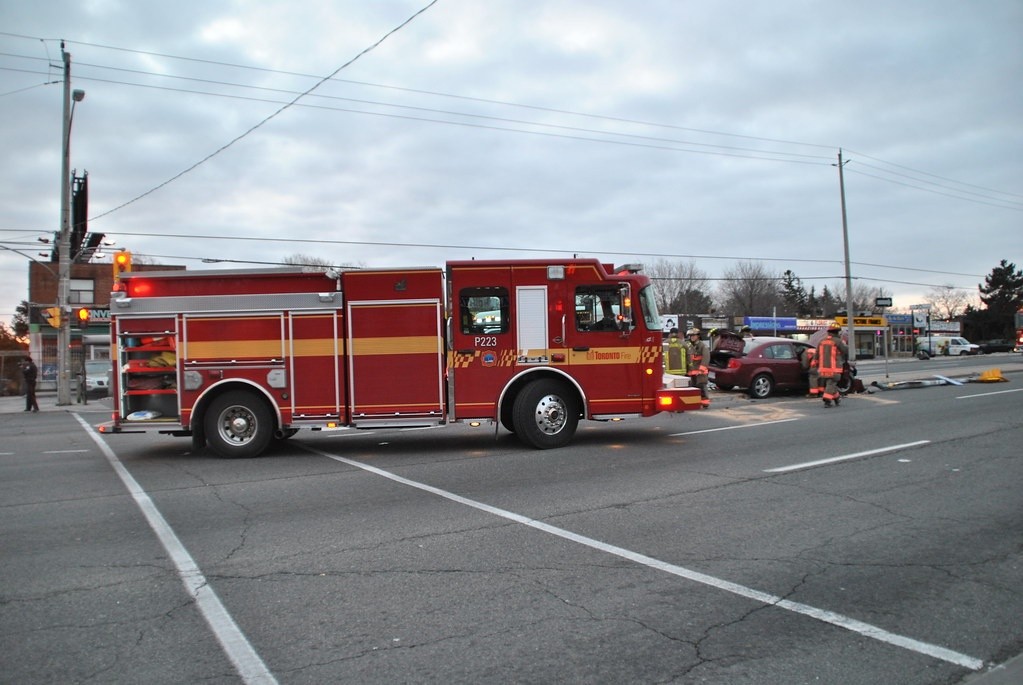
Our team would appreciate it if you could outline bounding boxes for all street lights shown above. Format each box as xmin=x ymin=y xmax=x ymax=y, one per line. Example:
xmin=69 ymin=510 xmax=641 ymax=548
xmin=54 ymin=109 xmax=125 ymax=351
xmin=830 ymin=154 xmax=856 ymax=361
xmin=55 ymin=85 xmax=87 ymax=407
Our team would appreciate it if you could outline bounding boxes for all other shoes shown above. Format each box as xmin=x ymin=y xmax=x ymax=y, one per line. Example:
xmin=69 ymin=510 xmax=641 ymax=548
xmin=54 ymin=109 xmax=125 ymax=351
xmin=824 ymin=398 xmax=831 ymax=406
xmin=703 ymin=404 xmax=708 ymax=408
xmin=32 ymin=409 xmax=38 ymax=412
xmin=24 ymin=409 xmax=31 ymax=411
xmin=836 ymin=402 xmax=841 ymax=406
xmin=806 ymin=393 xmax=818 ymax=398
xmin=819 ymin=392 xmax=823 ymax=396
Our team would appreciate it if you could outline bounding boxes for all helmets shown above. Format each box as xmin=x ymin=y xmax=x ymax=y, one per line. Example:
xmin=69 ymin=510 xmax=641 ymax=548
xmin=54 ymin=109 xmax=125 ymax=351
xmin=707 ymin=326 xmax=717 ymax=336
xmin=739 ymin=325 xmax=750 ymax=333
xmin=686 ymin=328 xmax=700 ymax=335
xmin=827 ymin=322 xmax=841 ymax=331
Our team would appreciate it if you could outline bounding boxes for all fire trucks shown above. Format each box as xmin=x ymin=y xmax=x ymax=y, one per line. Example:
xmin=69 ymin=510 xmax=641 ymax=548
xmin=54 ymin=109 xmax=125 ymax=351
xmin=94 ymin=254 xmax=715 ymax=458
xmin=1013 ymin=306 xmax=1023 ymax=353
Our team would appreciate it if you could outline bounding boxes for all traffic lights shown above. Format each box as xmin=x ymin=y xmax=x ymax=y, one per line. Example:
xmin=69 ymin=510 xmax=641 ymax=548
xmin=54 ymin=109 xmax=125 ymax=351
xmin=77 ymin=308 xmax=88 ymax=329
xmin=112 ymin=250 xmax=131 ymax=285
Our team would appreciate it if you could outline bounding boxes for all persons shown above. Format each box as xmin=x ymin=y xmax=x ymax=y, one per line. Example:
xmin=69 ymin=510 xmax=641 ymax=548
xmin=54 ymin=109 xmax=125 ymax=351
xmin=795 ymin=347 xmax=825 ymax=398
xmin=19 ymin=356 xmax=39 ymax=412
xmin=686 ymin=327 xmax=710 ymax=410
xmin=815 ymin=323 xmax=848 ymax=408
xmin=663 ymin=327 xmax=694 ymax=371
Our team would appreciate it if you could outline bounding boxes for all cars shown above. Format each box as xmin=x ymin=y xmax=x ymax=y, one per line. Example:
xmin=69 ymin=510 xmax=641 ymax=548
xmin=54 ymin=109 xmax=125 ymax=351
xmin=705 ymin=336 xmax=866 ymax=399
xmin=85 ymin=358 xmax=112 ymax=393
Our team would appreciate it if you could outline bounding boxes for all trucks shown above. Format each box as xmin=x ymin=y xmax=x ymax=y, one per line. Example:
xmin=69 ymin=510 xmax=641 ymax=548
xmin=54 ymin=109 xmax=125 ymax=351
xmin=921 ymin=337 xmax=982 ymax=356
